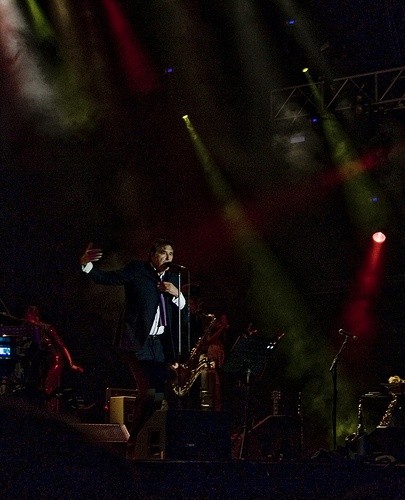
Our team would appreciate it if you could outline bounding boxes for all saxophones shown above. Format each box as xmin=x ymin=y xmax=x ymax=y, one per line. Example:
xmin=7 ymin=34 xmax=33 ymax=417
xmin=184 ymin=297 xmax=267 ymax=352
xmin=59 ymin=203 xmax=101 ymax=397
xmin=170 ymin=312 xmax=217 ymax=397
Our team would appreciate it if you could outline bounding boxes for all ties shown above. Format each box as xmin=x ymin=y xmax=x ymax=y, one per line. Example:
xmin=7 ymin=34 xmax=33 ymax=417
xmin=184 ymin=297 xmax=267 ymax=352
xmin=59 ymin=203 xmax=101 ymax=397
xmin=157 ymin=278 xmax=168 ymax=327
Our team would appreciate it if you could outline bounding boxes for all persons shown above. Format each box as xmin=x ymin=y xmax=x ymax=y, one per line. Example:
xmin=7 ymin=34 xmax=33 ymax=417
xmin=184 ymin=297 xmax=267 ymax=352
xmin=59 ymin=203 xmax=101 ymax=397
xmin=206 ymin=314 xmax=229 ymax=411
xmin=25 ymin=305 xmax=84 ymax=411
xmin=181 ymin=283 xmax=209 ymax=362
xmin=81 ymin=239 xmax=188 ymax=447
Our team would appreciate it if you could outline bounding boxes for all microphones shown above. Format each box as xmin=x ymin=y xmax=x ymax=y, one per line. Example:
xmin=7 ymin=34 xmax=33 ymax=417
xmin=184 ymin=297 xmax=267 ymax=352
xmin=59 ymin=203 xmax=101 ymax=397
xmin=337 ymin=329 xmax=358 ymax=341
xmin=164 ymin=262 xmax=186 ymax=269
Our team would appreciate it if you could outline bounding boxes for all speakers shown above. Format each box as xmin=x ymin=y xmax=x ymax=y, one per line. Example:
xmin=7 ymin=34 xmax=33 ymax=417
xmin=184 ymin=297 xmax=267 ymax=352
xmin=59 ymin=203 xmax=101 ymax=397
xmin=357 ymin=427 xmax=405 ymax=464
xmin=73 ymin=388 xmax=302 ymax=462
